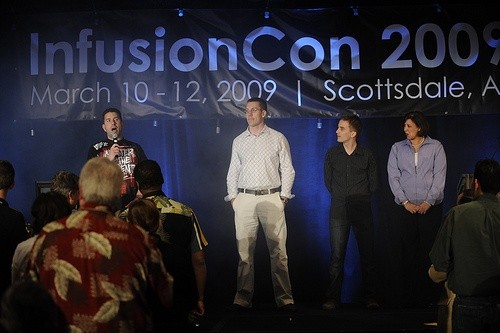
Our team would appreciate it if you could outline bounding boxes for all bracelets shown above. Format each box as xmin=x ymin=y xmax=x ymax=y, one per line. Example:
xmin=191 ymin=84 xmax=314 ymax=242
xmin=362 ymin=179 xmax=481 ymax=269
xmin=403 ymin=200 xmax=408 ymax=206
xmin=423 ymin=201 xmax=431 ymax=207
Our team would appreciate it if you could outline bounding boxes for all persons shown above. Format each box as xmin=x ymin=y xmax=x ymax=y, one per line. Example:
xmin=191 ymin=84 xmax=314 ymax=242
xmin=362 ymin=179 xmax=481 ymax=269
xmin=322 ymin=114 xmax=381 ymax=313
xmin=0 ymin=160 xmax=27 ymax=297
xmin=19 ymin=156 xmax=174 ymax=333
xmin=12 ymin=192 xmax=72 ymax=291
xmin=224 ymin=97 xmax=296 ymax=326
xmin=50 ymin=170 xmax=79 ymax=213
xmin=386 ymin=112 xmax=447 ymax=313
xmin=114 ymin=160 xmax=209 ymax=333
xmin=428 ymin=158 xmax=500 ymax=332
xmin=126 ymin=199 xmax=199 ymax=333
xmin=86 ymin=108 xmax=149 ymax=212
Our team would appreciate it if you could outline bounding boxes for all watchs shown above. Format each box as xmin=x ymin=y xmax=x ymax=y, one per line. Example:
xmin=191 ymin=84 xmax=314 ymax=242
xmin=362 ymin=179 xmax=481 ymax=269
xmin=281 ymin=197 xmax=289 ymax=204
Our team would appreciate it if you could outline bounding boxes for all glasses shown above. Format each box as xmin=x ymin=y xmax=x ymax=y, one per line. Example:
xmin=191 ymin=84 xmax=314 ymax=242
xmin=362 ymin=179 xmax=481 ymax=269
xmin=244 ymin=107 xmax=263 ymax=113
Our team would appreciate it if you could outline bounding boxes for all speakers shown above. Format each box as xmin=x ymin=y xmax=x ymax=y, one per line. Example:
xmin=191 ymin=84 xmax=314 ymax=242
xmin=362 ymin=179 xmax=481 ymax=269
xmin=35 ymin=181 xmax=55 ymax=202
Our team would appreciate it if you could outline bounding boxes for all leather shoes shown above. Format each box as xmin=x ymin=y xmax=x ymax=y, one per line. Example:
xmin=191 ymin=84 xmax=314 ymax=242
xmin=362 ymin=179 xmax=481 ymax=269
xmin=236 ymin=187 xmax=281 ymax=196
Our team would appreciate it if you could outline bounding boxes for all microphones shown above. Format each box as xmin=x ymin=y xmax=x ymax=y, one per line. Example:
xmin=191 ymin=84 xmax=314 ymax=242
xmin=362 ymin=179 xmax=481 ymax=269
xmin=112 ymin=134 xmax=119 ymax=160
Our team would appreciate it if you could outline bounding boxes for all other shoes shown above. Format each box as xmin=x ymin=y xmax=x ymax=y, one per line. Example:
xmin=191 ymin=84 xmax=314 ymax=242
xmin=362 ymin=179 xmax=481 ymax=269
xmin=395 ymin=299 xmax=419 ymax=311
xmin=364 ymin=296 xmax=379 ymax=310
xmin=278 ymin=304 xmax=297 ymax=313
xmin=228 ymin=304 xmax=254 ymax=314
xmin=322 ymin=299 xmax=341 ymax=311
xmin=424 ymin=300 xmax=436 ymax=312
